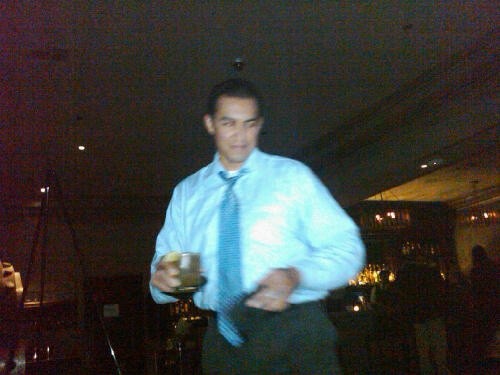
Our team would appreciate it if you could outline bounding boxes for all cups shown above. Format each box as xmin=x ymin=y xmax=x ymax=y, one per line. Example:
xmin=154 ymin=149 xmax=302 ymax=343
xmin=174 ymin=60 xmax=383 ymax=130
xmin=176 ymin=252 xmax=200 ymax=293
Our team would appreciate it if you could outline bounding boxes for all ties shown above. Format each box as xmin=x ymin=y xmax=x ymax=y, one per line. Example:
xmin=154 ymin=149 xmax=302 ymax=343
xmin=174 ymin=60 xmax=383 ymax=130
xmin=218 ymin=169 xmax=253 ymax=350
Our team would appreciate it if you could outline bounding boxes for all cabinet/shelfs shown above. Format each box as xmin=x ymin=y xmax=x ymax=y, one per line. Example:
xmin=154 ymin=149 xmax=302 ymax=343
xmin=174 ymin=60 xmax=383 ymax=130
xmin=343 ymin=201 xmax=456 ymax=318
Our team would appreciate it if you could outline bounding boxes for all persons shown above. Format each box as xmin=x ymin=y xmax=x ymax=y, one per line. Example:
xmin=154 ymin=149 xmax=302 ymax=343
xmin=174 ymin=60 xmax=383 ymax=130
xmin=149 ymin=78 xmax=367 ymax=375
xmin=370 ymin=246 xmax=500 ymax=375
